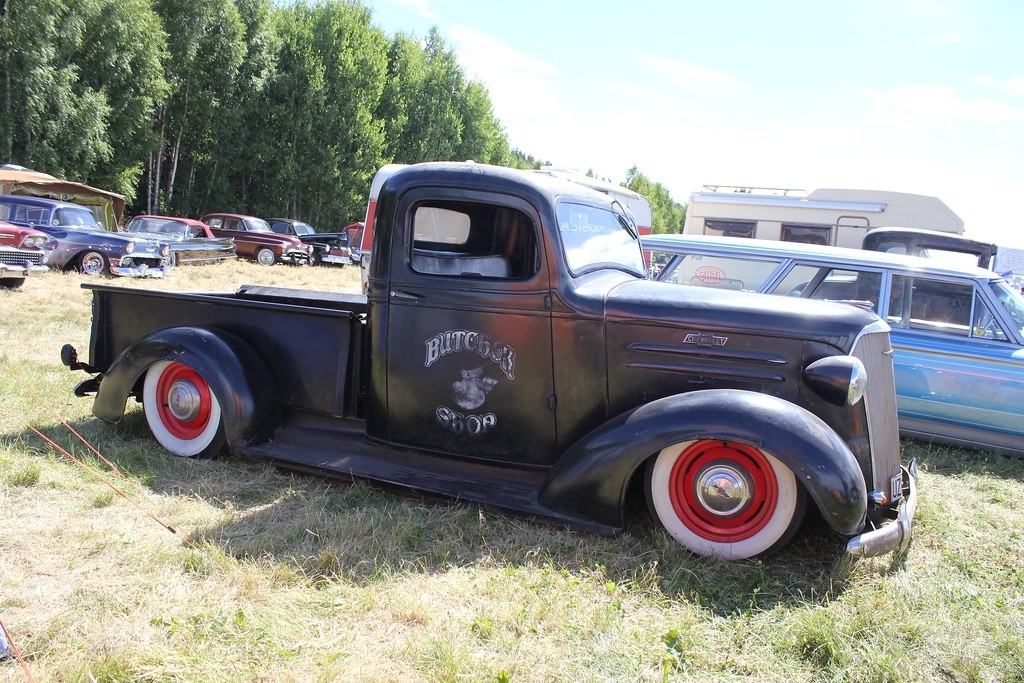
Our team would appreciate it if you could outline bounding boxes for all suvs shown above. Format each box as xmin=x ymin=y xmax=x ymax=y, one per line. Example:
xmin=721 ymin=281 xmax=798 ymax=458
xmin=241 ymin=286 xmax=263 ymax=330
xmin=0 ymin=194 xmax=176 ymax=278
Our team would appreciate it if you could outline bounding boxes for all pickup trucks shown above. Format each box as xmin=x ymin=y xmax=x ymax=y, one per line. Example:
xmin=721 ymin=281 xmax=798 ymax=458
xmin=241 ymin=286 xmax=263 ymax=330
xmin=60 ymin=161 xmax=919 ymax=571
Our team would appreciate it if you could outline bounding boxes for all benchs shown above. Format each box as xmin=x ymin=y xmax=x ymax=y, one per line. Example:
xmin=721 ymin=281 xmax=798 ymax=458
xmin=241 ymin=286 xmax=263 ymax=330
xmin=411 ymin=248 xmax=513 ymax=278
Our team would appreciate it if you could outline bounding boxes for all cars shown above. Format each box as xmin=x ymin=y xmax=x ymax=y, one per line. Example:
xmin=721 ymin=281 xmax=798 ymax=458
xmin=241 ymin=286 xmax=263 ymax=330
xmin=643 ymin=226 xmax=1024 ymax=462
xmin=123 ymin=215 xmax=215 ymax=241
xmin=261 ymin=218 xmax=365 ymax=269
xmin=0 ymin=221 xmax=49 ymax=288
xmin=187 ymin=212 xmax=316 ymax=267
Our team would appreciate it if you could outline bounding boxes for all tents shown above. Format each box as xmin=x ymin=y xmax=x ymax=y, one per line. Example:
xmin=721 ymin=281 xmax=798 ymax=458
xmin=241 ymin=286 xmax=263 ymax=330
xmin=0 ymin=163 xmax=125 ymax=232
xmin=342 ymin=222 xmax=365 ymax=247
